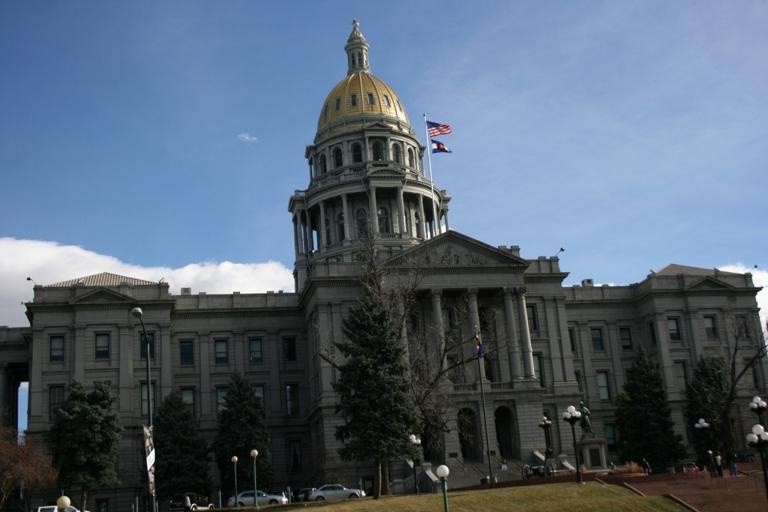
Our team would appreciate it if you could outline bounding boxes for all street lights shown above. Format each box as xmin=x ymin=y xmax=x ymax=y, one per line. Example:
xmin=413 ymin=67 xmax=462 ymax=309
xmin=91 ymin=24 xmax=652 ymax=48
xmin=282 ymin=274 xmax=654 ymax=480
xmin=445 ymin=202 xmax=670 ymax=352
xmin=539 ymin=416 xmax=552 ymax=458
xmin=563 ymin=405 xmax=583 ymax=484
xmin=409 ymin=434 xmax=421 ymax=494
xmin=132 ymin=307 xmax=157 ymax=512
xmin=695 ymin=418 xmax=713 ymax=454
xmin=57 ymin=496 xmax=71 ymax=512
xmin=250 ymin=449 xmax=259 ymax=509
xmin=746 ymin=425 xmax=768 ymax=502
xmin=749 ymin=396 xmax=767 ymax=426
xmin=436 ymin=465 xmax=450 ymax=512
xmin=231 ymin=456 xmax=238 ymax=508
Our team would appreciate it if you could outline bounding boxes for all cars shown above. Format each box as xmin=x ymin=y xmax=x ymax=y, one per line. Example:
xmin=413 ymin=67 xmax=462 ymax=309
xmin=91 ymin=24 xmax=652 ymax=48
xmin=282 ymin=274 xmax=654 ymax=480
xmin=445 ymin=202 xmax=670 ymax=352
xmin=298 ymin=484 xmax=367 ymax=502
xmin=37 ymin=505 xmax=81 ymax=512
xmin=228 ymin=491 xmax=288 ymax=508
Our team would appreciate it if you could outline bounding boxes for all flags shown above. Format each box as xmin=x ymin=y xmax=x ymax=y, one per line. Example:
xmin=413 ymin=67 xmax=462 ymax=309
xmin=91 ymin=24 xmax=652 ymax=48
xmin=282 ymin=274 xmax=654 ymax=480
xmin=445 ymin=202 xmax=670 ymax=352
xmin=431 ymin=139 xmax=453 ymax=155
xmin=476 ymin=335 xmax=485 ymax=358
xmin=426 ymin=121 xmax=452 ymax=138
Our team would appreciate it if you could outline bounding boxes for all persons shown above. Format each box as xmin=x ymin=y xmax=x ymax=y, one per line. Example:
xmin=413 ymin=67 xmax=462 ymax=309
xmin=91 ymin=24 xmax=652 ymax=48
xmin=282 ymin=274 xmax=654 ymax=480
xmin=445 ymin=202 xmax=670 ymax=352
xmin=641 ymin=457 xmax=650 ymax=475
xmin=707 ymin=449 xmax=717 ymax=477
xmin=608 ymin=462 xmax=616 ymax=470
xmin=578 ymin=401 xmax=595 ymax=440
xmin=715 ymin=452 xmax=723 ymax=479
xmin=727 ymin=447 xmax=737 ymax=476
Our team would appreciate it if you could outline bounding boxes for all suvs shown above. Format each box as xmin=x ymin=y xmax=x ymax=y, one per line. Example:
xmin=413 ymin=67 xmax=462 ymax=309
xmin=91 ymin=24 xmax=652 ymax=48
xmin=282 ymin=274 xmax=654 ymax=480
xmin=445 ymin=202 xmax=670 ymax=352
xmin=168 ymin=493 xmax=215 ymax=512
